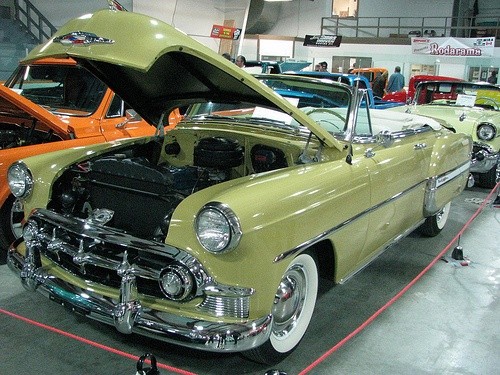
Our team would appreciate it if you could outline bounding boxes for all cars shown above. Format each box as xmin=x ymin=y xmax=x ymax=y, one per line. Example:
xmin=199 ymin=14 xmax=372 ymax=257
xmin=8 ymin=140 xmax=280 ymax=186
xmin=232 ymin=56 xmax=500 ymax=188
xmin=6 ymin=0 xmax=473 ymax=366
xmin=0 ymin=57 xmax=255 ymax=245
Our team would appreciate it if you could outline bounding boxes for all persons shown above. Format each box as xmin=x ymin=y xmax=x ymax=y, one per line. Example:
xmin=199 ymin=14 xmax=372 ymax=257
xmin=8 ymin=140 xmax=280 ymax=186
xmin=222 ymin=53 xmax=231 ymax=61
xmin=487 ymin=71 xmax=497 ymax=85
xmin=347 ymin=63 xmax=359 ymax=75
xmin=386 ymin=66 xmax=405 ymax=93
xmin=315 ymin=62 xmax=328 ymax=72
xmin=372 ymin=67 xmax=389 ymax=104
xmin=235 ymin=55 xmax=246 ymax=70
